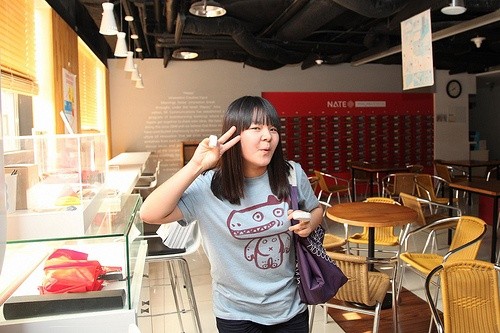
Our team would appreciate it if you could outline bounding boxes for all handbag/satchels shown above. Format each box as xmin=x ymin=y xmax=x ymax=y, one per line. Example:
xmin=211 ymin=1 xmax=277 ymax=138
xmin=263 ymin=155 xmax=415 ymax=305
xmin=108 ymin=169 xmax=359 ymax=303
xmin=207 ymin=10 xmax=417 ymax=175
xmin=291 ymin=219 xmax=349 ymax=305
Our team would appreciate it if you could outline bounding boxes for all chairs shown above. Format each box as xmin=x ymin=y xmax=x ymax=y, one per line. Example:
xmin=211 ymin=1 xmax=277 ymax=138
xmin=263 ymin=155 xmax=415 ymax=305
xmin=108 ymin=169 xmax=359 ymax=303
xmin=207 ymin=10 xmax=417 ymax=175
xmin=307 ymin=159 xmax=500 ymax=333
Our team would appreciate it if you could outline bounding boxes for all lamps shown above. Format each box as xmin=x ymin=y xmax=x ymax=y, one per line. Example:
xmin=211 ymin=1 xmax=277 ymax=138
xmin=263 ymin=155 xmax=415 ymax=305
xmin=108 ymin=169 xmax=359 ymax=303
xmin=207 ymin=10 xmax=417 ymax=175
xmin=441 ymin=0 xmax=467 ymax=15
xmin=188 ymin=0 xmax=226 ymax=18
xmin=171 ymin=47 xmax=198 ymax=59
xmin=99 ymin=0 xmax=143 ymax=88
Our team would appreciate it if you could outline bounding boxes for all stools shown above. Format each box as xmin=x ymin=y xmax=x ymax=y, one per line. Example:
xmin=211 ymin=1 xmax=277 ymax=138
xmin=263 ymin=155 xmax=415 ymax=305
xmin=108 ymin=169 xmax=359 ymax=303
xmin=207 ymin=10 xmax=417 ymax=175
xmin=133 ymin=160 xmax=202 ymax=333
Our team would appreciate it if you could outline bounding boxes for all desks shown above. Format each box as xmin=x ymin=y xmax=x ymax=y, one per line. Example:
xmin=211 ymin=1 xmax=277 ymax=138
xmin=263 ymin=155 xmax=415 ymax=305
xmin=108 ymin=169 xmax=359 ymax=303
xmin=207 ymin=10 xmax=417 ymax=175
xmin=352 ymin=163 xmax=409 ymax=203
xmin=327 ymin=202 xmax=419 ymax=310
xmin=436 ymin=160 xmax=500 ymax=206
xmin=449 ymin=179 xmax=500 ymax=263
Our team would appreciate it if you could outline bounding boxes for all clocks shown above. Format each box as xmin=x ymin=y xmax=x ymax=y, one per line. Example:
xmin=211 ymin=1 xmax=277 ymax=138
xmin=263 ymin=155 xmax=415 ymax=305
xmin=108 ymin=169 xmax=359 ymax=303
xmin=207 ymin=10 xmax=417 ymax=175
xmin=446 ymin=80 xmax=462 ymax=99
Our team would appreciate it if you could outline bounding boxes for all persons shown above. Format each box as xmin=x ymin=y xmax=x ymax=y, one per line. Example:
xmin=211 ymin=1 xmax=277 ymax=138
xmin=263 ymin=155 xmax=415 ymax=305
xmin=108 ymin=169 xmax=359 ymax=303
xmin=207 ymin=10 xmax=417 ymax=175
xmin=140 ymin=96 xmax=323 ymax=333
